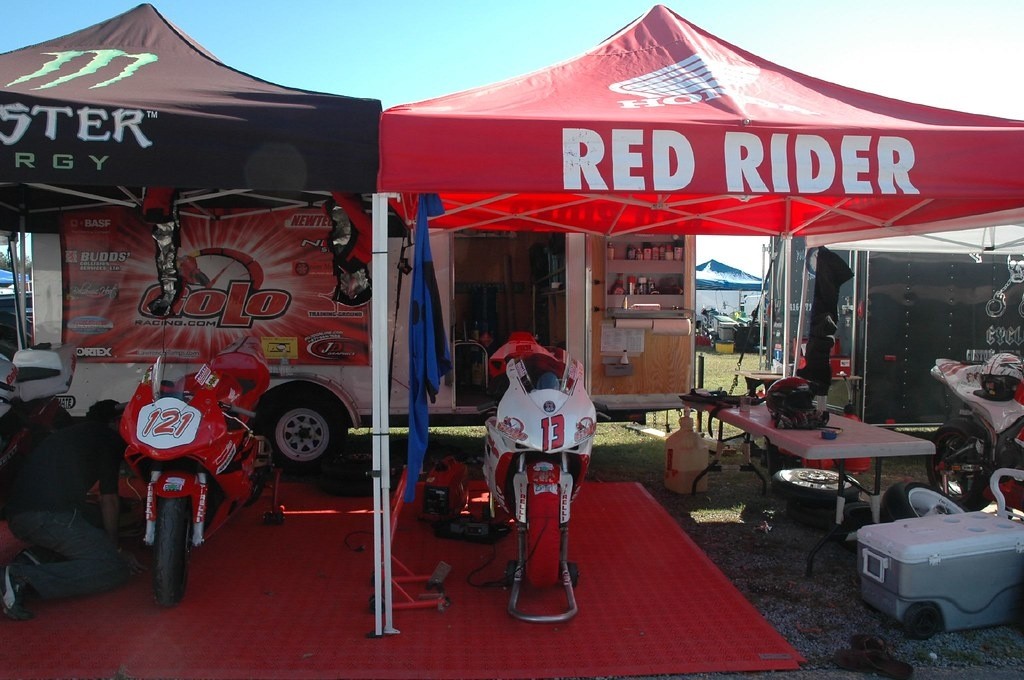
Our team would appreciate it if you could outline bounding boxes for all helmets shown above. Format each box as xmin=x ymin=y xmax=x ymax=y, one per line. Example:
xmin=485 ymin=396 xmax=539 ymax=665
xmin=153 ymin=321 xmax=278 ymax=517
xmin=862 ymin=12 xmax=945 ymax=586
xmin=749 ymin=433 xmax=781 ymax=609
xmin=0 ymin=353 xmax=18 ymax=420
xmin=766 ymin=377 xmax=818 ymax=429
xmin=979 ymin=352 xmax=1023 ymax=400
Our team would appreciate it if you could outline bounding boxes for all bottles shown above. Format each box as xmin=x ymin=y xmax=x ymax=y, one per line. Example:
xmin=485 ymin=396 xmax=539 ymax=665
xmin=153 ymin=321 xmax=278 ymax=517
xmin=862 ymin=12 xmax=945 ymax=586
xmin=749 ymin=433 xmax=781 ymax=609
xmin=665 ymin=417 xmax=707 ymax=493
xmin=623 ymin=295 xmax=628 ymax=310
xmin=612 ymin=273 xmax=625 ymax=295
xmin=626 ymin=242 xmax=684 ymax=261
xmin=607 ymin=242 xmax=614 ymax=259
xmin=471 ymin=321 xmax=479 ymax=341
xmin=647 ymin=276 xmax=655 ymax=295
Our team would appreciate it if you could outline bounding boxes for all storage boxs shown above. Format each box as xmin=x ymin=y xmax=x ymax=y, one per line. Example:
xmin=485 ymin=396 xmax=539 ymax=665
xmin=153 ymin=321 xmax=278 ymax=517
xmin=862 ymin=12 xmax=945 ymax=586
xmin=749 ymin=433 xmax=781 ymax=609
xmin=856 ymin=512 xmax=1024 ymax=640
xmin=719 ymin=327 xmax=733 ymax=340
xmin=715 ymin=341 xmax=734 ymax=353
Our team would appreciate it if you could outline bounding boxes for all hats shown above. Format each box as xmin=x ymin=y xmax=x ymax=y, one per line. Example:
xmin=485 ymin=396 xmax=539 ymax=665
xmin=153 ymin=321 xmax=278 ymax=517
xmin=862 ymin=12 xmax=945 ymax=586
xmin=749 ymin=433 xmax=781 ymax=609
xmin=86 ymin=399 xmax=125 ymax=423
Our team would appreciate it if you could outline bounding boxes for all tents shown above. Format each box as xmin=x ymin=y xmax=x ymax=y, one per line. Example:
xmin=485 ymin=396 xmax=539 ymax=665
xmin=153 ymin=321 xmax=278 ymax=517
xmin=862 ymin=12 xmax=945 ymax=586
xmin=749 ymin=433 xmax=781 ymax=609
xmin=2 ymin=0 xmax=1022 ymax=637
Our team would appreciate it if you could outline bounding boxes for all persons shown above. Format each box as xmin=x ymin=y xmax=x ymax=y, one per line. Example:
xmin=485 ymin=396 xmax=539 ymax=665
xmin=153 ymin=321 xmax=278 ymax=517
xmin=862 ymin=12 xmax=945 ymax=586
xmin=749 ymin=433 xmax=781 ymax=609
xmin=1 ymin=400 xmax=148 ymax=621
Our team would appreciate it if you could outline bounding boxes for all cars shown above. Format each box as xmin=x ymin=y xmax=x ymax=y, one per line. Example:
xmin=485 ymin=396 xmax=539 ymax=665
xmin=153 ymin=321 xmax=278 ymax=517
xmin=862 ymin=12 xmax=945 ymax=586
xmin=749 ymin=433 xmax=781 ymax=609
xmin=0 ymin=269 xmax=33 ymax=351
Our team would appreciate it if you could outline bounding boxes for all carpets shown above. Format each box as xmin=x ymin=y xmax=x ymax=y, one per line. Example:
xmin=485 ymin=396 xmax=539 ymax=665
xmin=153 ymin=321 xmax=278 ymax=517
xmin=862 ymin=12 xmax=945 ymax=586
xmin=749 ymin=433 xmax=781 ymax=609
xmin=0 ymin=483 xmax=805 ymax=680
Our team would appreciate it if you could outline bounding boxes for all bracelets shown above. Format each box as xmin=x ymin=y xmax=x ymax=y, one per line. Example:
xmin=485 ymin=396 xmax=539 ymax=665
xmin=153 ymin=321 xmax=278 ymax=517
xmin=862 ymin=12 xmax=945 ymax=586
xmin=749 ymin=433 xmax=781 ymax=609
xmin=118 ymin=547 xmax=122 ymax=553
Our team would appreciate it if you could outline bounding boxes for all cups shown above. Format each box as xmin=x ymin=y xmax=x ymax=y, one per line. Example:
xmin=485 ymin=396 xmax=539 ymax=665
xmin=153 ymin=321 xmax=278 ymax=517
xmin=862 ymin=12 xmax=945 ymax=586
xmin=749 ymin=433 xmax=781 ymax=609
xmin=817 ymin=396 xmax=827 ymax=411
xmin=740 ymin=396 xmax=751 ymax=412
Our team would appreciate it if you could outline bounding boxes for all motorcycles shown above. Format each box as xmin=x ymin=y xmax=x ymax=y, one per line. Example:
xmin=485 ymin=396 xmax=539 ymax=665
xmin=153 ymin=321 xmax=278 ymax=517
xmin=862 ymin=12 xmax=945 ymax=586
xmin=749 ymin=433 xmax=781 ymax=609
xmin=118 ymin=335 xmax=274 ymax=610
xmin=0 ymin=342 xmax=78 ymax=492
xmin=927 ymin=352 xmax=1024 ymax=517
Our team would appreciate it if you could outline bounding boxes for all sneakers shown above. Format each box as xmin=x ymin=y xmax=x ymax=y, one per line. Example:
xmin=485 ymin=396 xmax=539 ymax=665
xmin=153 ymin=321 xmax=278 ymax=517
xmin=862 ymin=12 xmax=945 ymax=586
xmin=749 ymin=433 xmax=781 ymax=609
xmin=0 ymin=547 xmax=42 ymax=621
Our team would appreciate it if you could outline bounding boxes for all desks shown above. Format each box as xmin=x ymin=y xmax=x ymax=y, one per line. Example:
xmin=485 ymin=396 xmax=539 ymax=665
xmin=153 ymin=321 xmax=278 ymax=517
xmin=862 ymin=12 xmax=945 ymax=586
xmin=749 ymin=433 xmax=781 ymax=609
xmin=736 ymin=371 xmax=783 ymax=396
xmin=680 ymin=397 xmax=936 ymax=580
xmin=714 ymin=315 xmax=734 ymax=332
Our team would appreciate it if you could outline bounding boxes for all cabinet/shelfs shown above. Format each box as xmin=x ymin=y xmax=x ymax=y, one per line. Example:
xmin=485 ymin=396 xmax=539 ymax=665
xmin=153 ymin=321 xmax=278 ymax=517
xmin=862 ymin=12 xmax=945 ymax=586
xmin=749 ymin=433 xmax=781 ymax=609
xmin=602 ymin=236 xmax=688 ymax=319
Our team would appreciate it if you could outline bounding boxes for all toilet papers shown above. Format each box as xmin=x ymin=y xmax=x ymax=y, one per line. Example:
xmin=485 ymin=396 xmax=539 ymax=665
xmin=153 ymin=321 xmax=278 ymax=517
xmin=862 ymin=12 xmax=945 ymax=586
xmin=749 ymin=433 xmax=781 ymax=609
xmin=614 ymin=319 xmax=652 ymax=329
xmin=652 ymin=319 xmax=691 ymax=336
